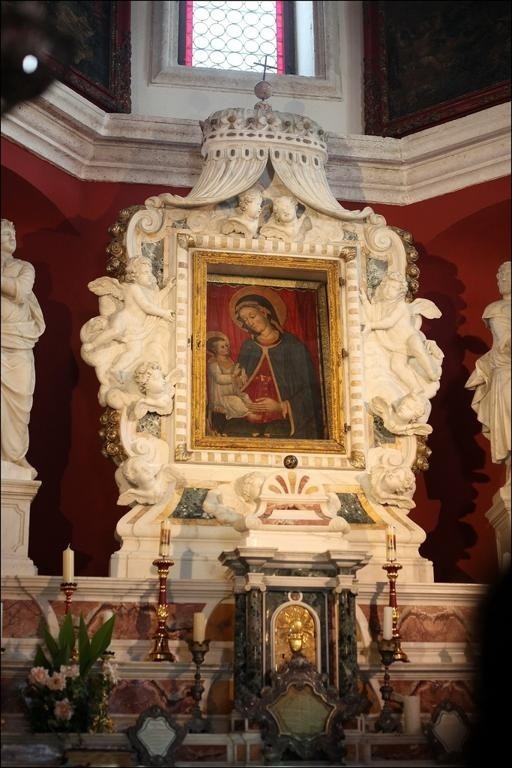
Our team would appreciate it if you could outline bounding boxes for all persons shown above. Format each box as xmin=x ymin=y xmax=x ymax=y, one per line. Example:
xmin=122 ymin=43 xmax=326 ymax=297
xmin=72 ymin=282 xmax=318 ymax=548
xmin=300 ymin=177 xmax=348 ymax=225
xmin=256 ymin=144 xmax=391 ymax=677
xmin=132 ymin=360 xmax=186 ymax=420
xmin=80 ymin=256 xmax=177 ymax=383
xmin=0 ymin=219 xmax=46 ymax=477
xmin=263 ymin=195 xmax=305 ymax=235
xmin=117 ymin=452 xmax=179 ymax=509
xmin=361 ymin=269 xmax=440 ymax=386
xmin=463 ymin=260 xmax=510 ymax=468
xmin=224 ymin=186 xmax=265 ymax=234
xmin=205 ymin=329 xmax=257 ymax=427
xmin=371 ymin=392 xmax=433 ymax=464
xmin=373 ymin=456 xmax=414 ymax=510
xmin=228 ymin=289 xmax=320 ymax=440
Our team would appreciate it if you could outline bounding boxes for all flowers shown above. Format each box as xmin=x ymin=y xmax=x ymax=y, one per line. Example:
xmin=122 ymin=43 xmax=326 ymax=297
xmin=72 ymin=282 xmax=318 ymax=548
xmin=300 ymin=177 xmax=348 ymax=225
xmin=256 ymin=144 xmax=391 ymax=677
xmin=19 ymin=611 xmax=117 ymax=733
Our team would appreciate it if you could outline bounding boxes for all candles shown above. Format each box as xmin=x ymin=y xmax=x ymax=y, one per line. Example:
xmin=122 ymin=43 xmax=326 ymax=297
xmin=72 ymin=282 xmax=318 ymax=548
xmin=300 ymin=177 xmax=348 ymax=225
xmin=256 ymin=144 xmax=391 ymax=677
xmin=386 ymin=526 xmax=397 ymax=562
xmin=192 ymin=612 xmax=205 ymax=645
xmin=62 ymin=546 xmax=74 ymax=581
xmin=158 ymin=519 xmax=171 ymax=557
xmin=382 ymin=606 xmax=394 ymax=640
xmin=403 ymin=693 xmax=421 ymax=732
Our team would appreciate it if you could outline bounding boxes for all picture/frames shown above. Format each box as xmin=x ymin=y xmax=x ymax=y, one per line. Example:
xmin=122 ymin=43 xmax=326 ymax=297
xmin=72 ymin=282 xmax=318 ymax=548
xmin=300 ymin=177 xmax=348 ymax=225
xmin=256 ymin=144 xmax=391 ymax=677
xmin=188 ymin=248 xmax=348 ymax=456
